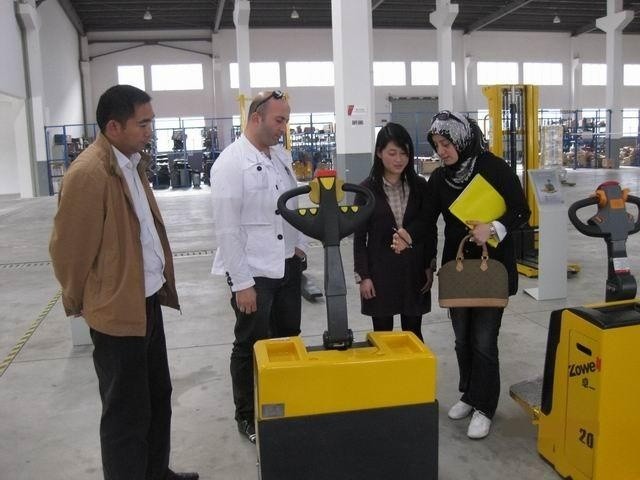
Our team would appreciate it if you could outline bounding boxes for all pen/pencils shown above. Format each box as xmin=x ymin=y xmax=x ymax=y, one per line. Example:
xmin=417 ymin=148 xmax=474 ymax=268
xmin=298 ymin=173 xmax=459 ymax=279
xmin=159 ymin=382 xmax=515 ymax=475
xmin=393 ymin=227 xmax=413 ymax=249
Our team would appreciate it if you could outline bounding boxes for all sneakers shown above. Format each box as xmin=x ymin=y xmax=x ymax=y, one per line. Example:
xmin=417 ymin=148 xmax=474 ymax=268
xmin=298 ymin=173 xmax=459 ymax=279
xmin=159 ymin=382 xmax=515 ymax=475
xmin=448 ymin=400 xmax=472 ymax=419
xmin=467 ymin=409 xmax=491 ymax=439
xmin=238 ymin=415 xmax=255 ymax=443
xmin=167 ymin=470 xmax=199 ymax=479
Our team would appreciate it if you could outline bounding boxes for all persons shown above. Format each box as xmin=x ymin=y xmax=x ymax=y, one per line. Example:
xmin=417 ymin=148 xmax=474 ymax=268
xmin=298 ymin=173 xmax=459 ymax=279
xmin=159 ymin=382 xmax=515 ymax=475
xmin=389 ymin=110 xmax=531 ymax=439
xmin=353 ymin=122 xmax=437 ymax=343
xmin=210 ymin=90 xmax=309 ymax=444
xmin=48 ymin=84 xmax=198 ymax=479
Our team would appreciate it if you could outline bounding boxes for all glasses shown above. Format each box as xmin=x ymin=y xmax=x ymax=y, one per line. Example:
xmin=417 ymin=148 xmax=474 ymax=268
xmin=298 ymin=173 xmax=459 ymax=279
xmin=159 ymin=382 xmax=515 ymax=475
xmin=430 ymin=110 xmax=467 ymax=133
xmin=255 ymin=89 xmax=286 ymax=114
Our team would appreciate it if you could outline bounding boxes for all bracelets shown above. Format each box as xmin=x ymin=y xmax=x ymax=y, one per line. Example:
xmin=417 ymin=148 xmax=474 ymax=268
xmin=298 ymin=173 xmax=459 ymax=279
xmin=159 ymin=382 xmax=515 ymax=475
xmin=489 ymin=222 xmax=497 ymax=240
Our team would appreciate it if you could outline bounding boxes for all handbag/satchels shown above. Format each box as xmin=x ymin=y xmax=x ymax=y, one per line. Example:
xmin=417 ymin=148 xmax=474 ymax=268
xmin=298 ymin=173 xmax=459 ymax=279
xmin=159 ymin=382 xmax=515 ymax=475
xmin=438 ymin=235 xmax=509 ymax=309
